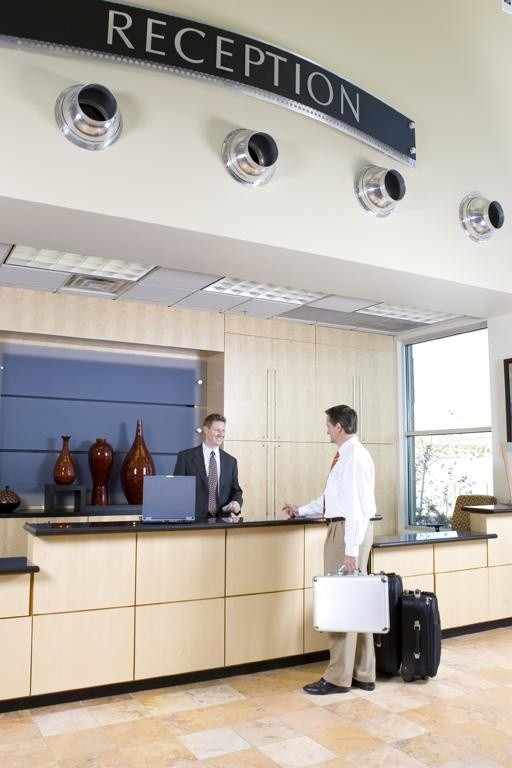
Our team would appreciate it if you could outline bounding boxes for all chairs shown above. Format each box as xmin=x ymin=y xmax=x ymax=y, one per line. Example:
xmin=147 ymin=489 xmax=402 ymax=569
xmin=427 ymin=495 xmax=496 ymax=532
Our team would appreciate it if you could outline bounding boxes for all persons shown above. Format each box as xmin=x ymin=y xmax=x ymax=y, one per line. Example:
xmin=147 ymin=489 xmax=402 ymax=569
xmin=172 ymin=413 xmax=243 ymax=519
xmin=280 ymin=403 xmax=379 ymax=695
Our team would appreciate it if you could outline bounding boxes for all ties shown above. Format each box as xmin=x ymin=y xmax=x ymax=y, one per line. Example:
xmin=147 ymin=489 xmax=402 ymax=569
xmin=208 ymin=452 xmax=219 ymax=518
xmin=322 ymin=451 xmax=342 ymax=526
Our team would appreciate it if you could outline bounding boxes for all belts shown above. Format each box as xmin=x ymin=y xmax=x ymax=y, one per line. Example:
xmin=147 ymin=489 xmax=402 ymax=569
xmin=325 ymin=517 xmax=344 ymax=525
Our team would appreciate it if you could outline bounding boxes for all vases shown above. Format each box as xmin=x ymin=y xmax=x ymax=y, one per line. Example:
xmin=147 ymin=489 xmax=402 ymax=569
xmin=0 ymin=486 xmax=21 ymax=511
xmin=89 ymin=438 xmax=113 ymax=505
xmin=120 ymin=419 xmax=155 ymax=505
xmin=54 ymin=435 xmax=76 ymax=485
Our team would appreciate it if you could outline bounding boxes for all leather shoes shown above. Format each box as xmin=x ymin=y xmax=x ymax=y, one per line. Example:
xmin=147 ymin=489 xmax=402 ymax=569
xmin=353 ymin=677 xmax=376 ymax=691
xmin=303 ymin=677 xmax=350 ymax=695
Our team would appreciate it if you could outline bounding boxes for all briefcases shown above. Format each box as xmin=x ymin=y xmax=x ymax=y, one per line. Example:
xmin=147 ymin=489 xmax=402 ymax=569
xmin=312 ymin=566 xmax=391 ymax=634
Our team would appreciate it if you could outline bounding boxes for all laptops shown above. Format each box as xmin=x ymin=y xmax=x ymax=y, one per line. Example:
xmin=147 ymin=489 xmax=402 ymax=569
xmin=139 ymin=475 xmax=196 ymax=523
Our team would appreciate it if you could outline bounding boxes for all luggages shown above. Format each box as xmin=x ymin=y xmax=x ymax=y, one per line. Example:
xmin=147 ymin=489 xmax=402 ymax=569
xmin=374 ymin=572 xmax=441 ymax=683
